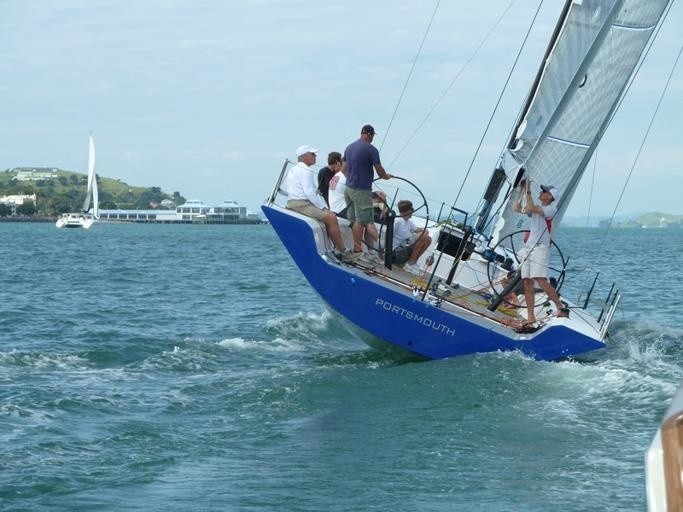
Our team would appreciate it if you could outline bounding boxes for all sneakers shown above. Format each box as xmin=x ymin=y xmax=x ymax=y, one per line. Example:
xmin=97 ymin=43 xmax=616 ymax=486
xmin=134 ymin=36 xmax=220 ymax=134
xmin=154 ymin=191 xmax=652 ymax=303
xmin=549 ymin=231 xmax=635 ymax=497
xmin=403 ymin=260 xmax=425 ymax=276
xmin=334 ymin=247 xmax=365 ymax=263
xmin=366 ymin=249 xmax=384 ymax=265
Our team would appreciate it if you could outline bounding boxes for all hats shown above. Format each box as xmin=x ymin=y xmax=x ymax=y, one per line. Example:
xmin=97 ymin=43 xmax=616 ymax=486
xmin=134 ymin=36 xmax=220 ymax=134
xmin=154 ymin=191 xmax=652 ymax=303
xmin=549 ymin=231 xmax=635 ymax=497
xmin=296 ymin=145 xmax=318 ymax=157
xmin=540 ymin=184 xmax=559 ymax=200
xmin=361 ymin=125 xmax=376 ymax=135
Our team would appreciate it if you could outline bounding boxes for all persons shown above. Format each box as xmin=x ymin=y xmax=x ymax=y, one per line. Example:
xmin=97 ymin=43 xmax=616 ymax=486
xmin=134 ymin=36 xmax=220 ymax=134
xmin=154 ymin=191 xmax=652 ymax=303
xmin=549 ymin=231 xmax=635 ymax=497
xmin=318 ymin=152 xmax=341 ymax=208
xmin=513 ymin=176 xmax=569 ymax=323
xmin=501 ymin=230 xmax=545 ymax=309
xmin=328 ymin=157 xmax=386 ymax=265
xmin=286 ymin=145 xmax=361 ymax=266
xmin=391 ymin=200 xmax=432 ymax=276
xmin=341 ymin=124 xmax=392 ymax=253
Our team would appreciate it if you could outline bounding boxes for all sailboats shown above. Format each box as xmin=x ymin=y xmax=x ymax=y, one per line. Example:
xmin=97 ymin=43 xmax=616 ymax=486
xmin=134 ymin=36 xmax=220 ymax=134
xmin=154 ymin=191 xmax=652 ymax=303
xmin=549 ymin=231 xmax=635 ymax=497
xmin=257 ymin=1 xmax=676 ymax=360
xmin=55 ymin=132 xmax=98 ymax=229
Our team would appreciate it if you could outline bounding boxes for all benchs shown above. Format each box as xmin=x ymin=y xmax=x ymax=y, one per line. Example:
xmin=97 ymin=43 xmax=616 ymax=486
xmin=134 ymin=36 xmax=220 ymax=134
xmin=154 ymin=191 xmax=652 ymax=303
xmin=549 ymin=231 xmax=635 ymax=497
xmin=323 ymin=211 xmax=356 ymax=253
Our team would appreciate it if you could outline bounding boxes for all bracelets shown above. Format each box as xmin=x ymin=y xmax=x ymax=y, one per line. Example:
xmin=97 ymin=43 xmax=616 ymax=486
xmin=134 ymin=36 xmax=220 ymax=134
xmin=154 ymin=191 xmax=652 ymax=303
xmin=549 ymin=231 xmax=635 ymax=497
xmin=526 ymin=191 xmax=531 ymax=194
